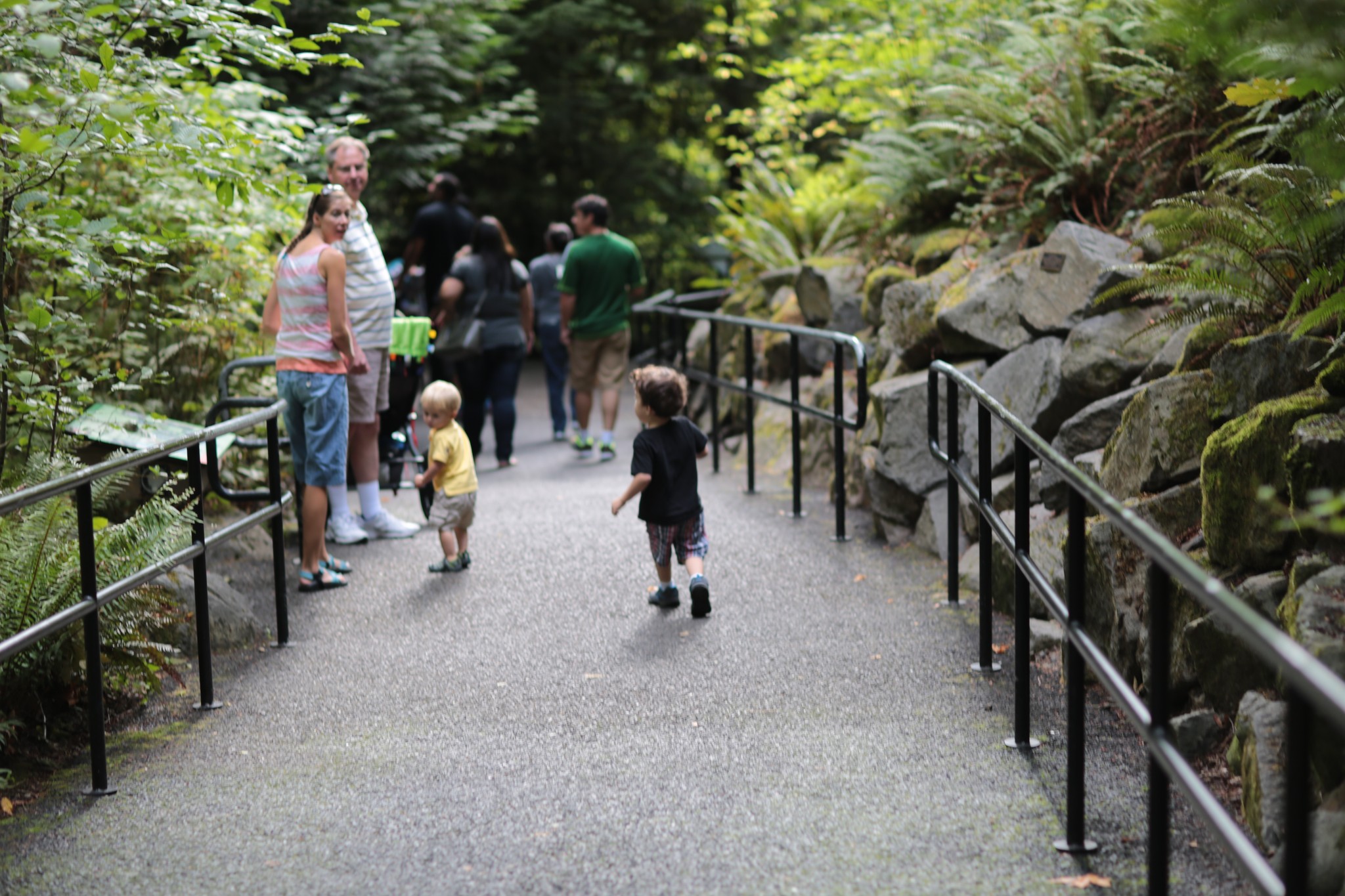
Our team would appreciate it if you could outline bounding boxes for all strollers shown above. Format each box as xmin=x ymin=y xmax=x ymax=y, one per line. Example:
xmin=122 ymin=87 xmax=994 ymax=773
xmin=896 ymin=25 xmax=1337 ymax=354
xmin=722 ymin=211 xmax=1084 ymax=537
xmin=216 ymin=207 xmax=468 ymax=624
xmin=376 ymin=309 xmax=444 ymax=519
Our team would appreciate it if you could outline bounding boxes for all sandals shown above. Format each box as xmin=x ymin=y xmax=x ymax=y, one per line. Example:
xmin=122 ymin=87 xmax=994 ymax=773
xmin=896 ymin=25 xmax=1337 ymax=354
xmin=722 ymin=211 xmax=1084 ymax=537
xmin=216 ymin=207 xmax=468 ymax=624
xmin=318 ymin=553 xmax=352 ymax=573
xmin=300 ymin=565 xmax=348 ymax=592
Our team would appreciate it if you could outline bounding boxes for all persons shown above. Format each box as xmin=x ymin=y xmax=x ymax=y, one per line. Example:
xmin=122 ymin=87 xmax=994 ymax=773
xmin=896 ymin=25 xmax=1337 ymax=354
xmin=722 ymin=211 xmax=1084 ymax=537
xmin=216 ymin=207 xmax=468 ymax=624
xmin=259 ymin=183 xmax=355 ymax=591
xmin=415 ymin=170 xmax=476 ymax=384
xmin=434 ymin=214 xmax=541 ymax=470
xmin=377 ymin=235 xmax=497 ymax=416
xmin=612 ymin=363 xmax=712 ymax=619
xmin=323 ymin=134 xmax=421 ymax=542
xmin=555 ymin=194 xmax=644 ymax=462
xmin=413 ymin=378 xmax=478 ymax=574
xmin=529 ymin=221 xmax=583 ymax=441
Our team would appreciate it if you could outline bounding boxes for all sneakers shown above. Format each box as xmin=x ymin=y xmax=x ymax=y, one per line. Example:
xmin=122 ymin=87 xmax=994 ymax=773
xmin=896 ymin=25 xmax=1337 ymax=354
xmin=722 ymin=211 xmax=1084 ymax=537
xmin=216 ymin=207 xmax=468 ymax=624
xmin=553 ymin=431 xmax=565 ymax=441
xmin=360 ymin=510 xmax=421 ymax=540
xmin=649 ymin=586 xmax=680 ymax=609
xmin=498 ymin=458 xmax=518 ymax=470
xmin=689 ymin=577 xmax=711 ymax=618
xmin=325 ymin=516 xmax=368 ymax=546
xmin=428 ymin=557 xmax=462 ymax=572
xmin=598 ymin=440 xmax=616 ymax=462
xmin=572 ymin=436 xmax=594 ymax=458
xmin=457 ymin=550 xmax=470 ymax=567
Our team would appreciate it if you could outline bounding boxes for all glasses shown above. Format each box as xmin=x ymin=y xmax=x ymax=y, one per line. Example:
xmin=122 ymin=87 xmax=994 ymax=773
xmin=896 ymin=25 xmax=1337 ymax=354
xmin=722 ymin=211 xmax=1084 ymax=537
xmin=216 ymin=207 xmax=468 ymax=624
xmin=316 ymin=183 xmax=344 ymax=200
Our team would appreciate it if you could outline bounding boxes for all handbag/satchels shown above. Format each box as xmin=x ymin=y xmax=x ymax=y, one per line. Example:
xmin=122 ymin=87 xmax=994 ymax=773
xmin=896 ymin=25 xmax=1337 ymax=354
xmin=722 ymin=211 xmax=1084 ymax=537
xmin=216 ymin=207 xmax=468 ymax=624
xmin=431 ymin=314 xmax=487 ymax=359
